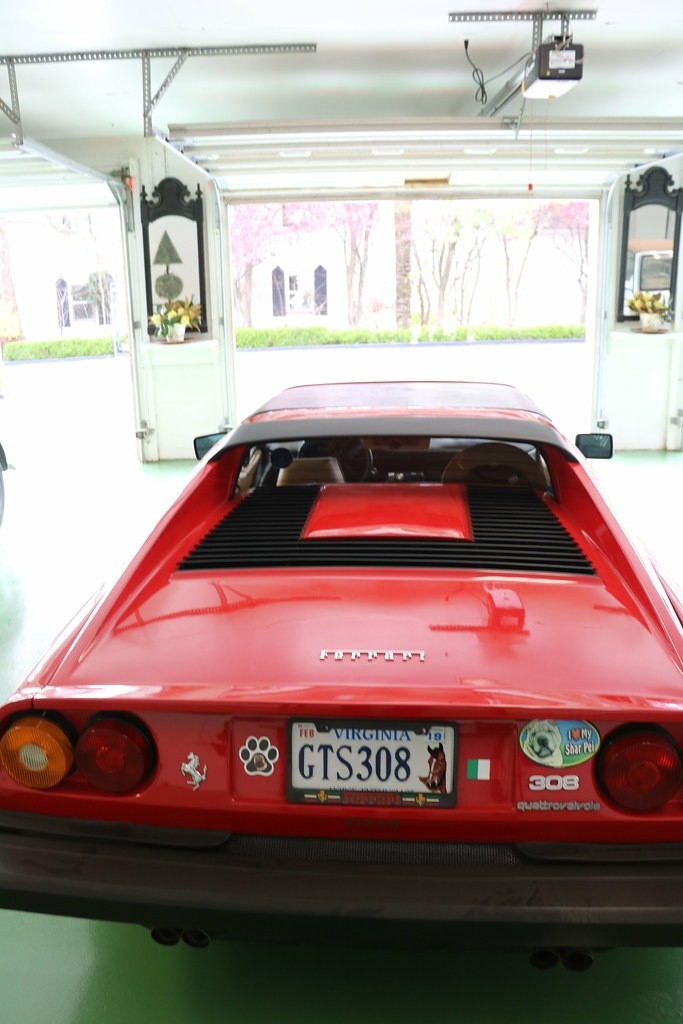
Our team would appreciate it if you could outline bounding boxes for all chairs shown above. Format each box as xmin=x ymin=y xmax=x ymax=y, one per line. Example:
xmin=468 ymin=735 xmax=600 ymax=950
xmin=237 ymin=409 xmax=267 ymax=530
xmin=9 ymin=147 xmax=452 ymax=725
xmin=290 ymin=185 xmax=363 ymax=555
xmin=444 ymin=443 xmax=547 ymax=484
xmin=275 ymin=455 xmax=353 ymax=487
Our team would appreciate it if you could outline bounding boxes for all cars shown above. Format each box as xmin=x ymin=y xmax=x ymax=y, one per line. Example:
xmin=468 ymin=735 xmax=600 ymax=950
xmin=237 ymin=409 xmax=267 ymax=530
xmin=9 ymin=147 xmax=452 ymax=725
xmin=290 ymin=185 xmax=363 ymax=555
xmin=0 ymin=383 xmax=683 ymax=971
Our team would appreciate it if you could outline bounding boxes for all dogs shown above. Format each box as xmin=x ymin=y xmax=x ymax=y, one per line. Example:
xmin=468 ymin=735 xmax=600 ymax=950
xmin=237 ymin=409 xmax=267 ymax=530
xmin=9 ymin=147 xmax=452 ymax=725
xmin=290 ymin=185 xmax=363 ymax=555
xmin=419 ymin=742 xmax=448 ymax=794
xmin=524 ymin=719 xmax=563 ymax=768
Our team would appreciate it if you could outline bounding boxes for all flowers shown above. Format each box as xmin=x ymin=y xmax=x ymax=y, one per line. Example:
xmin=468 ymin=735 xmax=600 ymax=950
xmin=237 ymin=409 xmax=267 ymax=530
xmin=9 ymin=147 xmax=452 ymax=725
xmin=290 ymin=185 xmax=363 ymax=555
xmin=628 ymin=291 xmax=672 ymax=322
xmin=148 ymin=301 xmax=201 ymax=331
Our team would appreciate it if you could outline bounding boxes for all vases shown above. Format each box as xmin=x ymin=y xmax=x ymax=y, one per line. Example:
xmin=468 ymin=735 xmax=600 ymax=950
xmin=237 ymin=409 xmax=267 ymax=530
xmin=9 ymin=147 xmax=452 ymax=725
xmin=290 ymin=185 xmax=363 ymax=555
xmin=166 ymin=322 xmax=187 ymax=343
xmin=637 ymin=313 xmax=662 ymax=331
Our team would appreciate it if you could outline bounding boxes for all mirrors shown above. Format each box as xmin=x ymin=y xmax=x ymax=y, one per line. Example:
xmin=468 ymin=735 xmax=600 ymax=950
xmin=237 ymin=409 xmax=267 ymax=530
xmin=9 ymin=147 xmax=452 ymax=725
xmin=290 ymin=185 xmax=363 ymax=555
xmin=138 ymin=176 xmax=208 ymax=339
xmin=616 ymin=166 xmax=683 ymax=322
